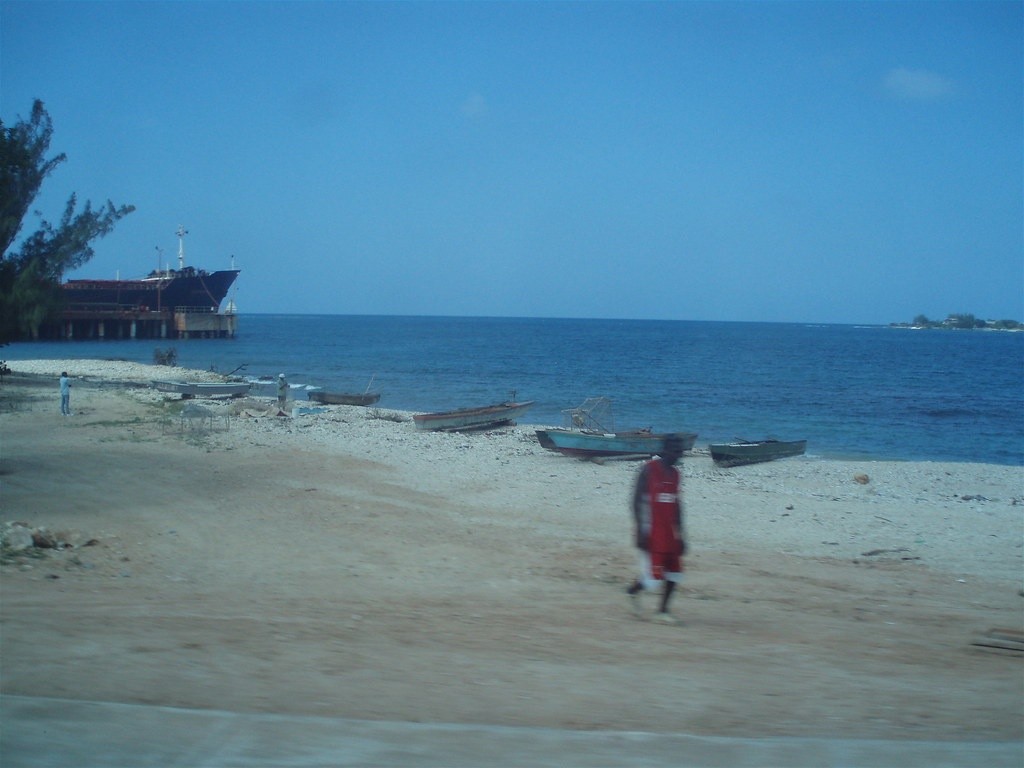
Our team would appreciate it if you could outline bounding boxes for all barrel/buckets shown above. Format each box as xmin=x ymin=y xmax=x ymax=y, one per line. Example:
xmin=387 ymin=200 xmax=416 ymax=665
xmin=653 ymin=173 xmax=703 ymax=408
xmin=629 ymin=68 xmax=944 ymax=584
xmin=292 ymin=409 xmax=300 ymax=419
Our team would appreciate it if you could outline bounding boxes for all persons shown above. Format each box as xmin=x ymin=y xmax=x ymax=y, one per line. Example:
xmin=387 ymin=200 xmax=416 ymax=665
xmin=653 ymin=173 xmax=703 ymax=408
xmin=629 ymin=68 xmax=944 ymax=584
xmin=60 ymin=372 xmax=74 ymax=417
xmin=276 ymin=373 xmax=290 ymax=413
xmin=625 ymin=431 xmax=688 ymax=627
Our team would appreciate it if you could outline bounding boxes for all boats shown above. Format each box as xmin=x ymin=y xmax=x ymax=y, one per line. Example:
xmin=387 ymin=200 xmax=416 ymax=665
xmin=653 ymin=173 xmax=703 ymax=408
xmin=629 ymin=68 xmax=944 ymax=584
xmin=708 ymin=440 xmax=808 ymax=468
xmin=415 ymin=402 xmax=536 ymax=431
xmin=306 ymin=389 xmax=382 ymax=407
xmin=538 ymin=419 xmax=698 ymax=458
xmin=152 ymin=379 xmax=251 ymax=394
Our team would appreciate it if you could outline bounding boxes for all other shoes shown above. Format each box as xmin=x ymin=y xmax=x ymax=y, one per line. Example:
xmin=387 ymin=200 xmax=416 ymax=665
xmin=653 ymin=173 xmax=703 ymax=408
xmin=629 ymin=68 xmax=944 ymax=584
xmin=624 ymin=588 xmax=640 ymax=604
xmin=62 ymin=412 xmax=74 ymax=417
xmin=654 ymin=609 xmax=676 ymax=623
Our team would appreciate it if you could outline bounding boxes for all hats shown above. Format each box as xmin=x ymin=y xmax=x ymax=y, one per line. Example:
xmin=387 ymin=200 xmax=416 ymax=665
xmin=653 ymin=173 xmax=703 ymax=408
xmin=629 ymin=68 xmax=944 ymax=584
xmin=279 ymin=373 xmax=286 ymax=378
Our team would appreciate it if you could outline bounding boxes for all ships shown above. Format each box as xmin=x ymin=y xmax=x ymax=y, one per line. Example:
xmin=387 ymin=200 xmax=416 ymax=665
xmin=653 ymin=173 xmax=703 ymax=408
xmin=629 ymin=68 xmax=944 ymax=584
xmin=45 ymin=220 xmax=241 ymax=313
